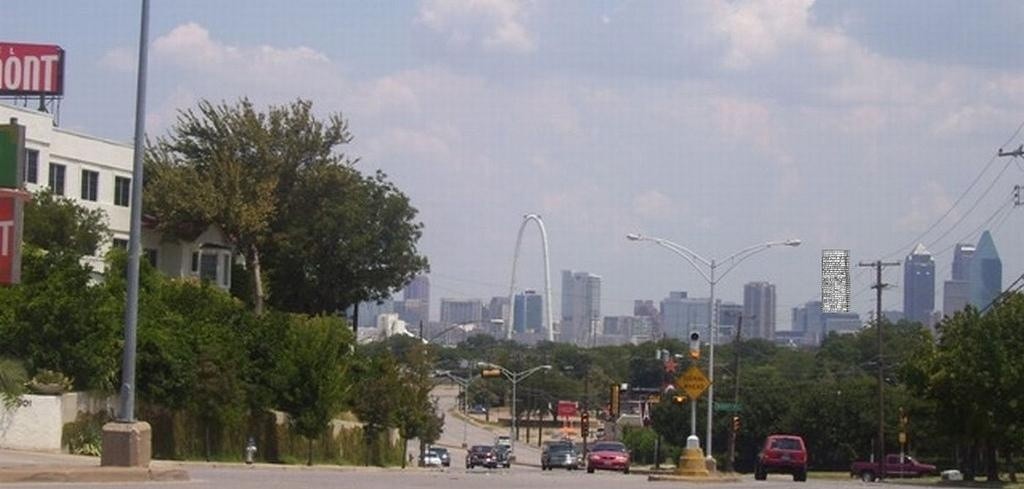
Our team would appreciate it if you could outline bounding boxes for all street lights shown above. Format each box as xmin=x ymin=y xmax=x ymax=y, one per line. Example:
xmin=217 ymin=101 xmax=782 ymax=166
xmin=417 ymin=317 xmax=506 ymax=465
xmin=438 ymin=368 xmax=483 ymax=449
xmin=624 ymin=228 xmax=803 ymax=476
xmin=476 ymin=361 xmax=553 ymax=443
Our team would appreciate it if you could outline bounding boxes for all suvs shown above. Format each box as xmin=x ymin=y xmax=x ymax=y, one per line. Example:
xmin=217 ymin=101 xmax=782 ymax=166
xmin=540 ymin=442 xmax=580 ymax=472
xmin=754 ymin=434 xmax=810 ymax=482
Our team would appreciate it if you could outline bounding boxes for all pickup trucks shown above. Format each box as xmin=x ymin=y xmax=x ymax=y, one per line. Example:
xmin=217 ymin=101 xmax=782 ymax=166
xmin=849 ymin=453 xmax=939 ymax=485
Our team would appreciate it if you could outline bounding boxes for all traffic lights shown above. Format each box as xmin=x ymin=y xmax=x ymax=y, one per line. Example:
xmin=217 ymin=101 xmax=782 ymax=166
xmin=687 ymin=329 xmax=703 ymax=362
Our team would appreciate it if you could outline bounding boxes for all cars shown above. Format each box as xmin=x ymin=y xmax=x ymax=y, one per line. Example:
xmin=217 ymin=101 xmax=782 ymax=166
xmin=584 ymin=439 xmax=635 ymax=475
xmin=415 ymin=446 xmax=451 ymax=469
xmin=465 ymin=434 xmax=514 ymax=469
